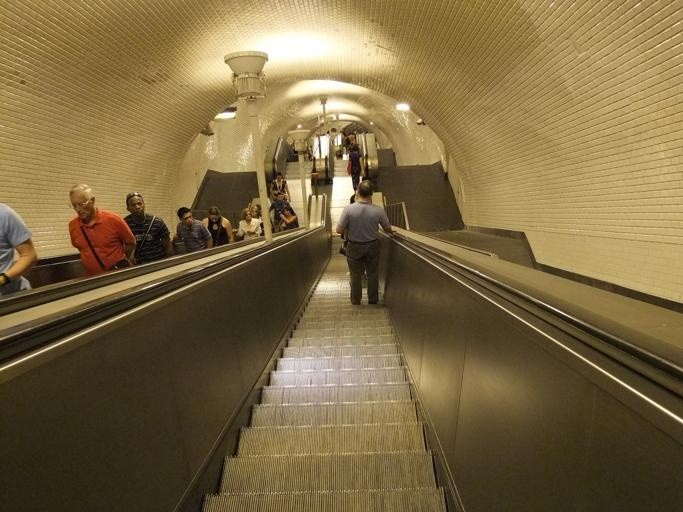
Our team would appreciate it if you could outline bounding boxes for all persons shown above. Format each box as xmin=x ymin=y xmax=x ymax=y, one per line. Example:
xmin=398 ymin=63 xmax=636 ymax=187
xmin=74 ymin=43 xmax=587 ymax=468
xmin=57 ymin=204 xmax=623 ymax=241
xmin=199 ymin=206 xmax=235 ymax=248
xmin=0 ymin=202 xmax=37 ymax=296
xmin=170 ymin=206 xmax=213 ymax=254
xmin=66 ymin=182 xmax=138 ymax=276
xmin=248 ymin=172 xmax=298 ymax=236
xmin=335 ymin=126 xmax=397 ymax=307
xmin=232 ymin=207 xmax=262 ymax=242
xmin=122 ymin=191 xmax=173 ymax=269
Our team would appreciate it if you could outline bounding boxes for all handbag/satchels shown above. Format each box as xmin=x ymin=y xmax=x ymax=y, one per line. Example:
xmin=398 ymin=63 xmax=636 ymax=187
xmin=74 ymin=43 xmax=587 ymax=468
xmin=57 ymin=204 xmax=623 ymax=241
xmin=347 ymin=161 xmax=351 ymax=176
xmin=106 ymin=259 xmax=129 ymax=272
xmin=339 ymin=240 xmax=348 ymax=256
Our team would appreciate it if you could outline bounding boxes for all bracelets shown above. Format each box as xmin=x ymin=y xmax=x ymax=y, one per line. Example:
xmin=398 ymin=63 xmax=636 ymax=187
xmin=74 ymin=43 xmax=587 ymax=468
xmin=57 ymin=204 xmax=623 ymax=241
xmin=0 ymin=273 xmax=10 ymax=285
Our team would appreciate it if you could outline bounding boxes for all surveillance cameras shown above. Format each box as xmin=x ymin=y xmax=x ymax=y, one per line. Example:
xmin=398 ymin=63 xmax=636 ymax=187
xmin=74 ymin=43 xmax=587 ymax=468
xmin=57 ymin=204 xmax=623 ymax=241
xmin=416 ymin=119 xmax=426 ymax=125
xmin=201 ymin=125 xmax=215 ymax=136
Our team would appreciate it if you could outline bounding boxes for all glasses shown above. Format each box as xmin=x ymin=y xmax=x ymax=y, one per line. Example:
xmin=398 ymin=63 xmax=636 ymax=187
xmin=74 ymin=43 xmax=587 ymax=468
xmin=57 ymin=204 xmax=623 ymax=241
xmin=69 ymin=199 xmax=90 ymax=209
xmin=126 ymin=192 xmax=141 ymax=199
